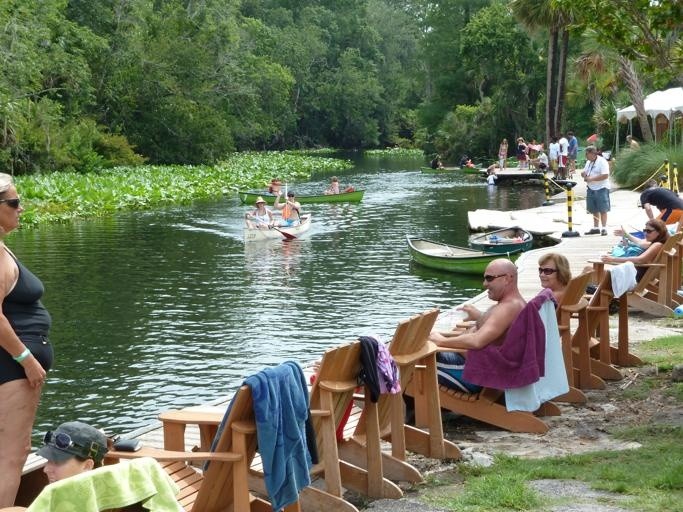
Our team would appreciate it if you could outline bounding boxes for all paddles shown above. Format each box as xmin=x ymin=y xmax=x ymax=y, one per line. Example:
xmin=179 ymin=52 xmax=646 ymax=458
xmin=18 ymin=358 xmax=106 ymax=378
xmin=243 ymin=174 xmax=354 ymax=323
xmin=249 ymin=214 xmax=295 ymax=238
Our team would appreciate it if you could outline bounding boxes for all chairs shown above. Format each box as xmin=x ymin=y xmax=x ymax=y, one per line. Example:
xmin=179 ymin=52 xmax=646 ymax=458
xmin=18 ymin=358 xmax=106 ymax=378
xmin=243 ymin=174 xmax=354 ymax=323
xmin=102 ymin=384 xmax=304 ymax=512
xmin=188 ymin=341 xmax=405 ymax=512
xmin=339 ymin=308 xmax=465 ymax=483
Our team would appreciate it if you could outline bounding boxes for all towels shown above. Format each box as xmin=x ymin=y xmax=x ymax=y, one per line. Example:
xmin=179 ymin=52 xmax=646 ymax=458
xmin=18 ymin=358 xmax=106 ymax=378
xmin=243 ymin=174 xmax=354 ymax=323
xmin=200 ymin=359 xmax=312 ymax=512
xmin=24 ymin=455 xmax=188 ymax=512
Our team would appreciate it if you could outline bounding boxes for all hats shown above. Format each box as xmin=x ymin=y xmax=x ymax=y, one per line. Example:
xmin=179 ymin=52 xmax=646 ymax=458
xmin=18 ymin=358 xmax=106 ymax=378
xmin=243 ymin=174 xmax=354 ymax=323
xmin=35 ymin=420 xmax=109 ymax=463
xmin=517 ymin=137 xmax=524 ymax=142
xmin=255 ymin=196 xmax=266 ymax=204
xmin=288 ymin=190 xmax=294 ymax=196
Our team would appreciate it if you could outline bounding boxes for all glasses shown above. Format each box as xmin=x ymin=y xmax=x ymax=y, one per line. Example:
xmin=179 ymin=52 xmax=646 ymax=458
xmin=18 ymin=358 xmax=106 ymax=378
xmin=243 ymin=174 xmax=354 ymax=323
xmin=288 ymin=195 xmax=294 ymax=197
xmin=643 ymin=229 xmax=655 ymax=233
xmin=480 ymin=274 xmax=512 ymax=281
xmin=0 ymin=199 xmax=20 ymax=208
xmin=539 ymin=268 xmax=558 ymax=275
xmin=43 ymin=430 xmax=89 ymax=451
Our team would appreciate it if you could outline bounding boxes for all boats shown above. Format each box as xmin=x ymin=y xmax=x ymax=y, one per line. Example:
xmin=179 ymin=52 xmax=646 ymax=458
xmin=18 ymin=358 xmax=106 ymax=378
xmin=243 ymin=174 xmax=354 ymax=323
xmin=241 ymin=213 xmax=311 ymax=246
xmin=237 ymin=189 xmax=365 ymax=206
xmin=419 ymin=163 xmax=484 ymax=174
xmin=403 ymin=225 xmax=535 ymax=280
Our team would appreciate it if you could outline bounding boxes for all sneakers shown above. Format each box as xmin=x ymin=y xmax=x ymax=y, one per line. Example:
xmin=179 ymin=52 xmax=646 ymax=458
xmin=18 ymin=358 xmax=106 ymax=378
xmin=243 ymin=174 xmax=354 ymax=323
xmin=602 ymin=229 xmax=608 ymax=235
xmin=584 ymin=229 xmax=600 ymax=234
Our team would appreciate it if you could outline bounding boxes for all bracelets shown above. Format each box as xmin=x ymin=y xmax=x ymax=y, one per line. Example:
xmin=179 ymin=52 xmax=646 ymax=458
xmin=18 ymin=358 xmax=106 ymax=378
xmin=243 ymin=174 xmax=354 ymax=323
xmin=13 ymin=347 xmax=30 ymax=363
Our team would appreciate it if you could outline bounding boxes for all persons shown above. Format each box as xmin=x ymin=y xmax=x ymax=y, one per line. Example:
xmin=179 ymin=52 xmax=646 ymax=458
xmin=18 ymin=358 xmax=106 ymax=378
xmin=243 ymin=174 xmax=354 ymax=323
xmin=245 ymin=176 xmax=339 ymax=231
xmin=428 ymin=258 xmax=527 ymax=394
xmin=460 ymin=155 xmax=469 ymax=169
xmin=538 ymin=253 xmax=572 ymax=302
xmin=486 ymin=131 xmax=578 ymax=185
xmin=581 ymin=136 xmax=683 ymax=286
xmin=0 ymin=172 xmax=53 ymax=512
xmin=35 ymin=421 xmax=109 ymax=484
xmin=432 ymin=154 xmax=441 ymax=169
